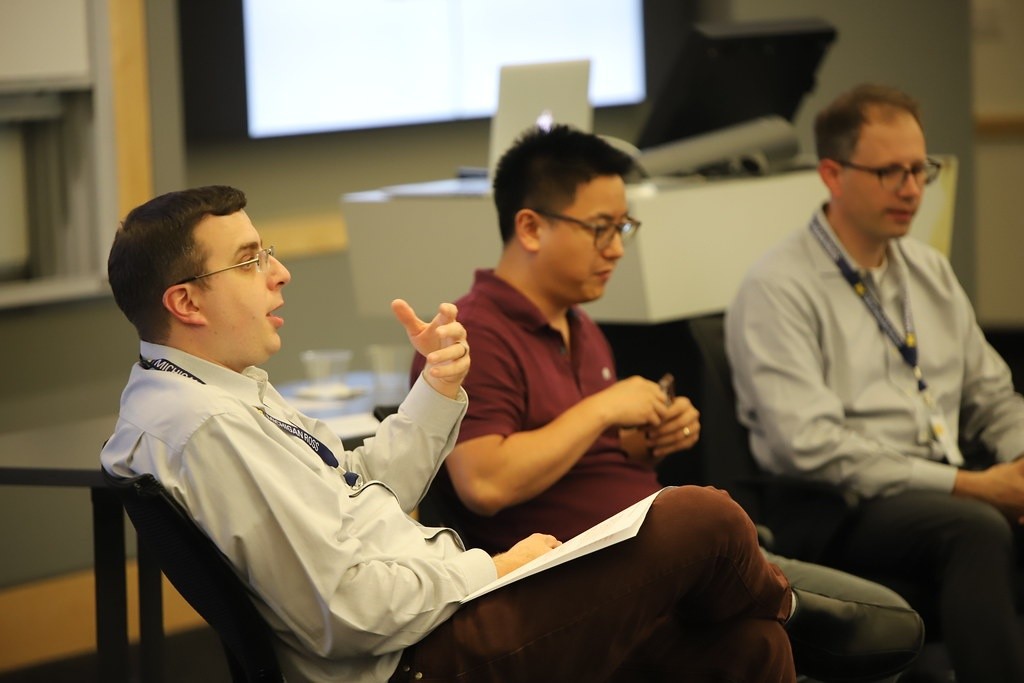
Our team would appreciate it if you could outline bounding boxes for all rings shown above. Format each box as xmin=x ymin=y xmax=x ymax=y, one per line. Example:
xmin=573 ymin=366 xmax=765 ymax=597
xmin=455 ymin=342 xmax=468 ymax=359
xmin=683 ymin=427 xmax=690 ymax=438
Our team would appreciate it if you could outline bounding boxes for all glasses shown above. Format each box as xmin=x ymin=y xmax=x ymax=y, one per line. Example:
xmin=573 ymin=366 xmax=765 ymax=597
xmin=533 ymin=206 xmax=644 ymax=251
xmin=177 ymin=243 xmax=278 ymax=285
xmin=841 ymin=154 xmax=945 ymax=193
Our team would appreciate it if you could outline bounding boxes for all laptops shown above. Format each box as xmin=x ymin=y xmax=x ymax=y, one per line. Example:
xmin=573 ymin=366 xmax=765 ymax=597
xmin=383 ymin=56 xmax=593 ymax=202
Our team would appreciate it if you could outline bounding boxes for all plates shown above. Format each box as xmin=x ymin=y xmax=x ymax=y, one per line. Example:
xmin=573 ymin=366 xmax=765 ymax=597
xmin=297 ymin=389 xmax=360 ymax=400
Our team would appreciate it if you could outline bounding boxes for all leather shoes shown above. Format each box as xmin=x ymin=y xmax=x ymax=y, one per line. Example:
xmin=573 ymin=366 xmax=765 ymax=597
xmin=784 ymin=582 xmax=926 ymax=682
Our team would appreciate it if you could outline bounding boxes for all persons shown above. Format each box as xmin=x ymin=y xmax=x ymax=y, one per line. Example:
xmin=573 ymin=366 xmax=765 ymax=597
xmin=99 ymin=187 xmax=925 ymax=683
xmin=722 ymin=83 xmax=1023 ymax=683
xmin=408 ymin=124 xmax=914 ymax=679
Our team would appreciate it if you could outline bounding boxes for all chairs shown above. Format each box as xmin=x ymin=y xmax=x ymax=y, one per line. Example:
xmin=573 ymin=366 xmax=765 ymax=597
xmin=94 ymin=463 xmax=318 ymax=682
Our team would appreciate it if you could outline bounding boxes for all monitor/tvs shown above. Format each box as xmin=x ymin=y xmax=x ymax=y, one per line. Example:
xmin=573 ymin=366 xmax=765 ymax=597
xmin=633 ymin=15 xmax=841 ymax=150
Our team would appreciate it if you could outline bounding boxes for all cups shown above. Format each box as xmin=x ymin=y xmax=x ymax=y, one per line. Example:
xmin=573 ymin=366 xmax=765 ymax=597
xmin=301 ymin=348 xmax=354 ymax=393
xmin=364 ymin=348 xmax=411 ymax=408
